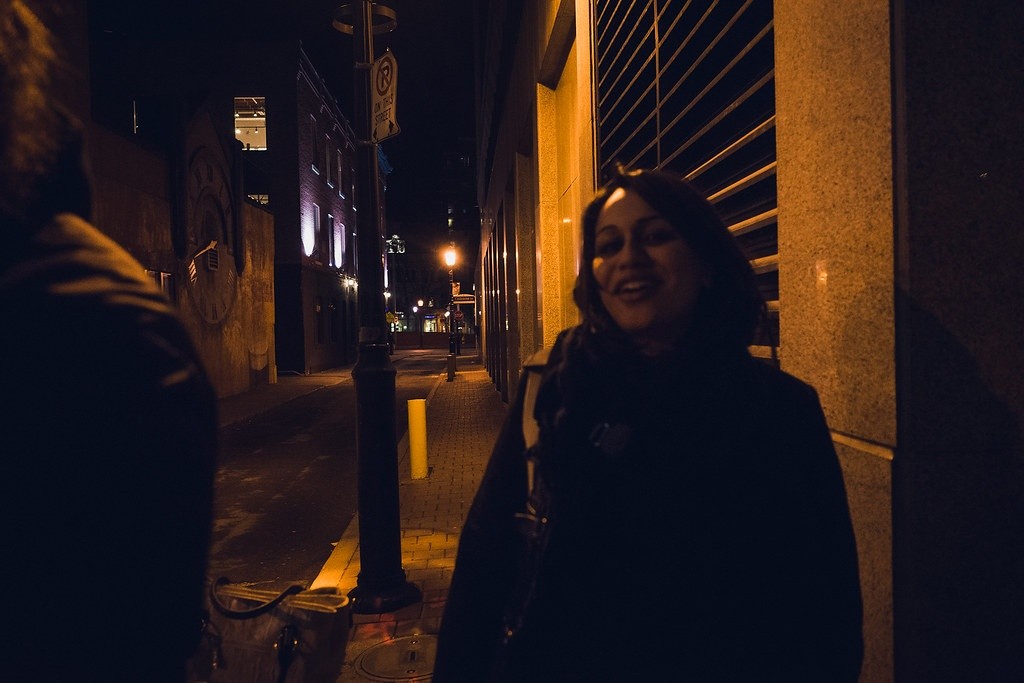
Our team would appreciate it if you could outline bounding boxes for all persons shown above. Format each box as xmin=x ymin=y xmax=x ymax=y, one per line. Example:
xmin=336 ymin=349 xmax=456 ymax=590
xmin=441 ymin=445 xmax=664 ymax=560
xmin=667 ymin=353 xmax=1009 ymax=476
xmin=0 ymin=1 xmax=215 ymax=683
xmin=433 ymin=170 xmax=864 ymax=683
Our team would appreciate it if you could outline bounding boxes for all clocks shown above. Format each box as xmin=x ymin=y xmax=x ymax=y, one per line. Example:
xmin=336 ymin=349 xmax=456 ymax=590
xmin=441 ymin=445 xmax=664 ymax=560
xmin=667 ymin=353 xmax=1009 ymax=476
xmin=181 ymin=146 xmax=237 ymax=327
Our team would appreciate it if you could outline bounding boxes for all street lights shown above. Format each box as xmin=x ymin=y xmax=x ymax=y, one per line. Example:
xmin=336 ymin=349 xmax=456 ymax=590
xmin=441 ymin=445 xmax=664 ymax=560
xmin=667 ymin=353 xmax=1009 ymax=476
xmin=412 ymin=299 xmax=424 ymax=348
xmin=445 ymin=246 xmax=458 ymax=373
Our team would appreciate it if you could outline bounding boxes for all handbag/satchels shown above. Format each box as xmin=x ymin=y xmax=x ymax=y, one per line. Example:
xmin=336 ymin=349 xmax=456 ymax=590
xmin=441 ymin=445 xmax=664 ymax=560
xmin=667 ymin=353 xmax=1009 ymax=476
xmin=191 ymin=575 xmax=351 ymax=683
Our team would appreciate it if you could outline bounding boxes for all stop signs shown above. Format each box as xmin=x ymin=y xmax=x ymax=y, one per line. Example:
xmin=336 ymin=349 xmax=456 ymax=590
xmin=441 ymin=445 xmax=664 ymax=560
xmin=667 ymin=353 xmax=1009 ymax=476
xmin=455 ymin=311 xmax=464 ymax=322
